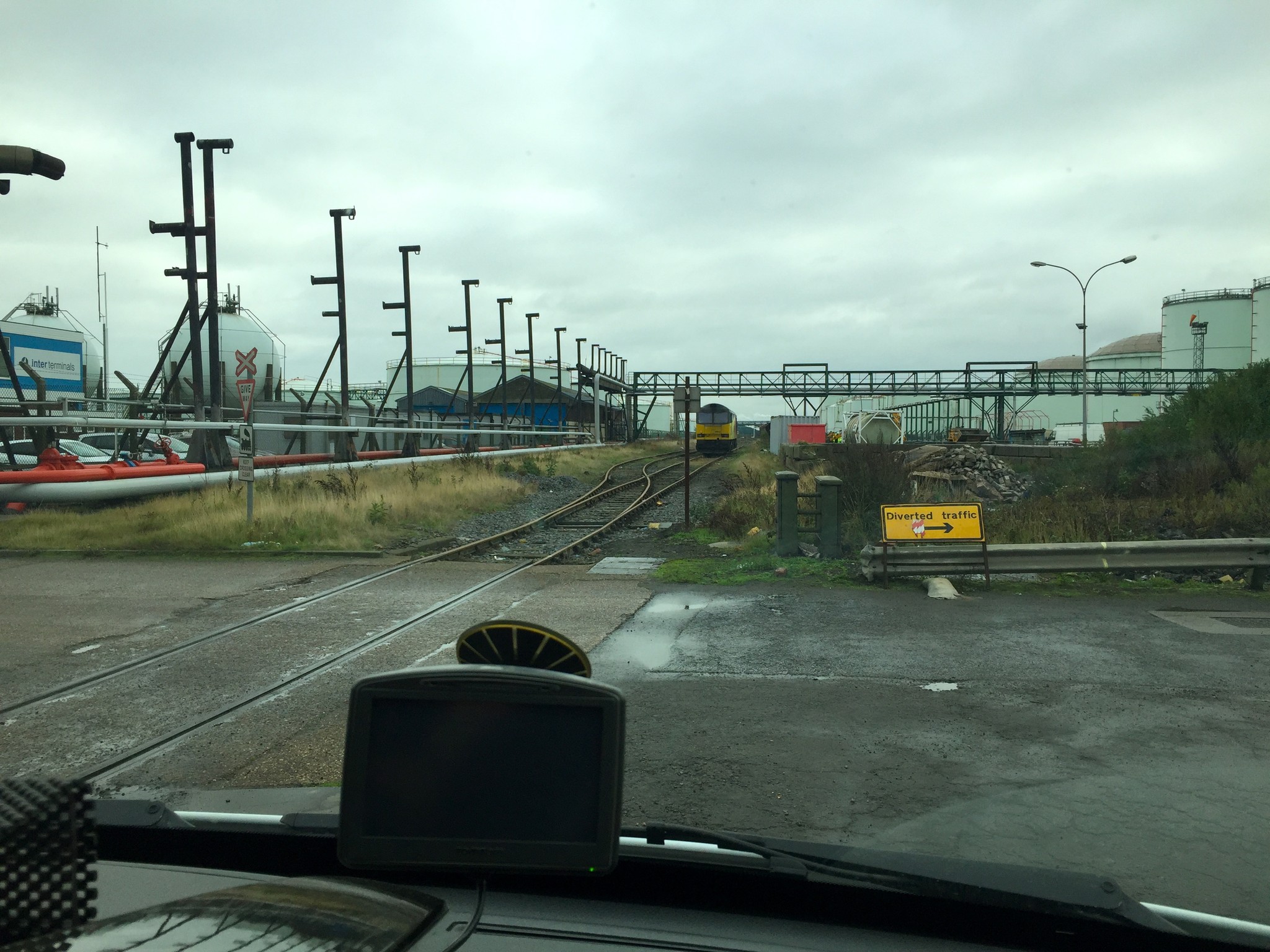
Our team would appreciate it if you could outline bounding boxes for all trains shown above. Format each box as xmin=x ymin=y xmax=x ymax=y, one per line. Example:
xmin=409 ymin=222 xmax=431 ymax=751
xmin=696 ymin=403 xmax=738 ymax=455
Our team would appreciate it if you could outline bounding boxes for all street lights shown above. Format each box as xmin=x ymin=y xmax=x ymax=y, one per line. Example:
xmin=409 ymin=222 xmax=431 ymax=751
xmin=1030 ymin=255 xmax=1139 ymax=450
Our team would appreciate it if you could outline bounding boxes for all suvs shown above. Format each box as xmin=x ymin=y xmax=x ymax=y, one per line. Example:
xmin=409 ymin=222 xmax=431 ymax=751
xmin=77 ymin=432 xmax=194 ymax=463
xmin=168 ymin=432 xmax=283 ymax=470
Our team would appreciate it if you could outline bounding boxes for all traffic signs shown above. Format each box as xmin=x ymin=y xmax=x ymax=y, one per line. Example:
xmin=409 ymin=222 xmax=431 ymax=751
xmin=881 ymin=501 xmax=987 ymax=544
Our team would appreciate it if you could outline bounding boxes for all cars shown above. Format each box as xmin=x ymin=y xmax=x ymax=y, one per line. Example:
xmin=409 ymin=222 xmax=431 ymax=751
xmin=438 ymin=437 xmax=470 ymax=448
xmin=0 ymin=439 xmax=123 ymax=472
xmin=436 ymin=440 xmax=452 ymax=449
